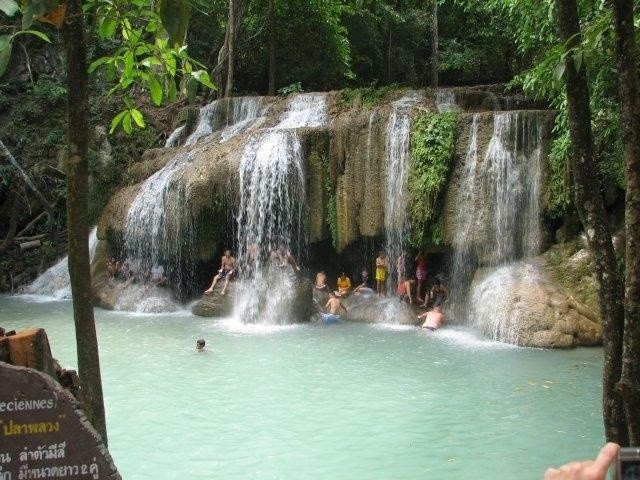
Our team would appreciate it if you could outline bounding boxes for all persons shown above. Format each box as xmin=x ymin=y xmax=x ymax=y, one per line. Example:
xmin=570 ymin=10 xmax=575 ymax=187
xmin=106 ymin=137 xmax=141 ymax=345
xmin=106 ymin=257 xmax=169 ymax=289
xmin=238 ymin=239 xmax=301 ymax=278
xmin=375 ymin=247 xmax=429 ymax=305
xmin=205 ymin=251 xmax=237 ymax=296
xmin=318 ymin=290 xmax=348 ymax=324
xmin=353 ymin=269 xmax=375 ymax=296
xmin=314 ymin=269 xmax=330 ymax=298
xmin=418 ymin=280 xmax=450 ymax=311
xmin=543 ymin=442 xmax=620 ymax=480
xmin=333 ymin=269 xmax=350 ymax=297
xmin=195 ymin=339 xmax=207 ymax=352
xmin=418 ymin=306 xmax=446 ymax=333
